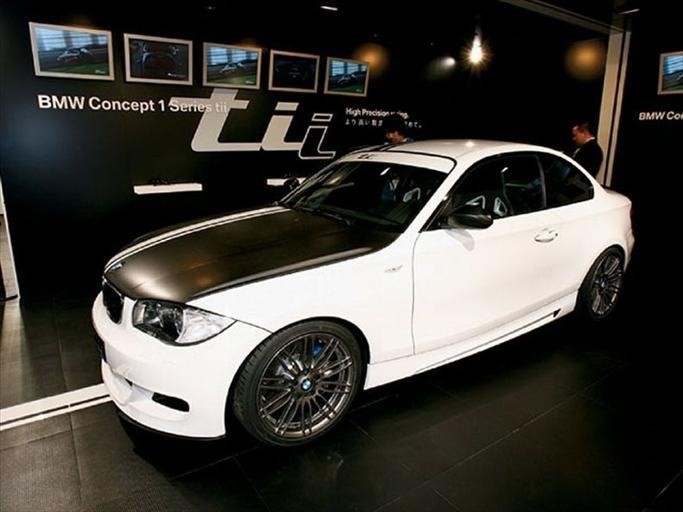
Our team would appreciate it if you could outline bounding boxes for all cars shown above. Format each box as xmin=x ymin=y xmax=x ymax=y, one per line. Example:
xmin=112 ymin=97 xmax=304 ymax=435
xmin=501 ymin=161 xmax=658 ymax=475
xmin=56 ymin=46 xmax=96 ymax=66
xmin=337 ymin=73 xmax=359 ymax=87
xmin=219 ymin=62 xmax=254 ymax=77
xmin=90 ymin=139 xmax=634 ymax=446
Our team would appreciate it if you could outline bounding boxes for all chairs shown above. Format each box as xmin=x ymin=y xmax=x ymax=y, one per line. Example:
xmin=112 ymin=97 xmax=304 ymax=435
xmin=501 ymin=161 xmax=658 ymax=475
xmin=385 ymin=190 xmax=423 ymax=224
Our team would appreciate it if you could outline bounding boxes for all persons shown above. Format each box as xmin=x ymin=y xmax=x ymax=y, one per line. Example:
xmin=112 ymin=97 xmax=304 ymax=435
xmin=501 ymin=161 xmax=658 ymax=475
xmin=572 ymin=124 xmax=603 ymax=180
xmin=381 ymin=113 xmax=414 ymax=144
